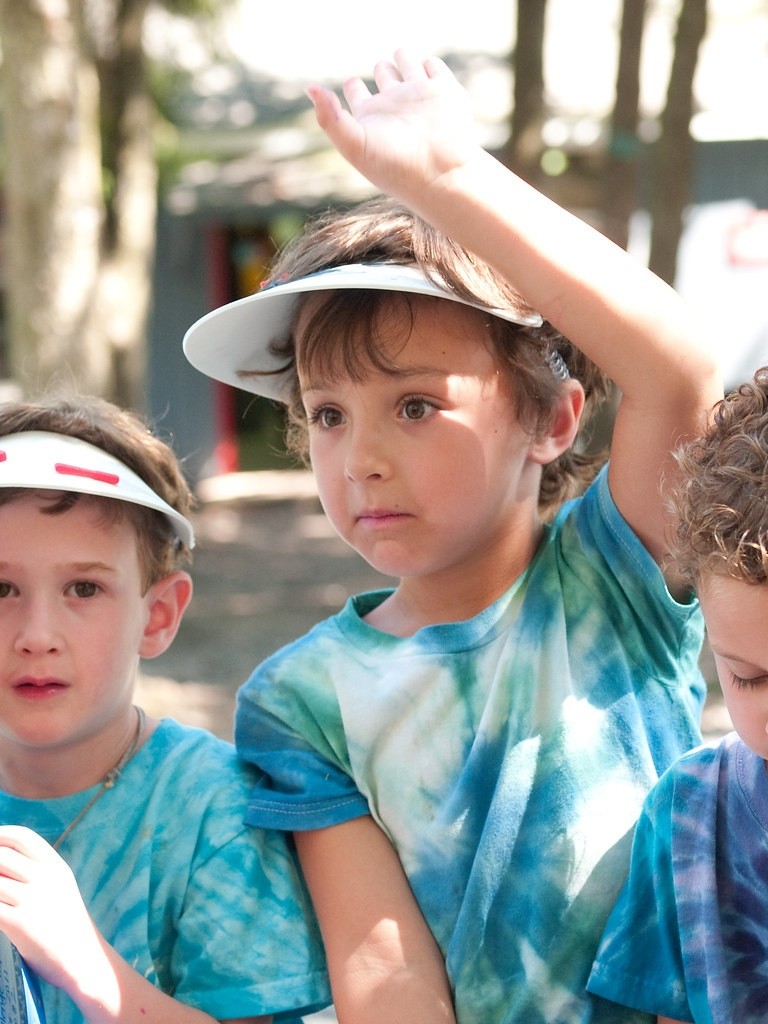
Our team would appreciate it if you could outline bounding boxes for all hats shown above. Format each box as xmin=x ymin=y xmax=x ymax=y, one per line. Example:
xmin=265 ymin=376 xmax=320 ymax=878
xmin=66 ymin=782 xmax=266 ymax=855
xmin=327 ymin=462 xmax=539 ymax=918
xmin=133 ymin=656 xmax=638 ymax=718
xmin=183 ymin=253 xmax=542 ymax=405
xmin=0 ymin=431 xmax=195 ymax=551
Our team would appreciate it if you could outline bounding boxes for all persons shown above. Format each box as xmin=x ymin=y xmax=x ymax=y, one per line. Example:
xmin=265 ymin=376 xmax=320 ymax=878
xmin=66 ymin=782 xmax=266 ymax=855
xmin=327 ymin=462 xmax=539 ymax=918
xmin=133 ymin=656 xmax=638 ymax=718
xmin=229 ymin=44 xmax=734 ymax=1024
xmin=0 ymin=388 xmax=334 ymax=1024
xmin=583 ymin=365 xmax=768 ymax=1024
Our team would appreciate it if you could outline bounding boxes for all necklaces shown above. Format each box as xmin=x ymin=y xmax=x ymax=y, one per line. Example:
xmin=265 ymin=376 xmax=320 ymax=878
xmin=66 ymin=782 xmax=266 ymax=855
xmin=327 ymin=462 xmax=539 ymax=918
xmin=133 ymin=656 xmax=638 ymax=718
xmin=49 ymin=702 xmax=147 ymax=853
